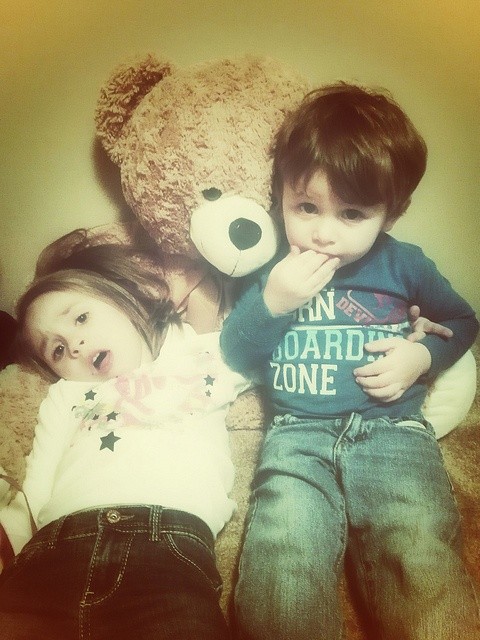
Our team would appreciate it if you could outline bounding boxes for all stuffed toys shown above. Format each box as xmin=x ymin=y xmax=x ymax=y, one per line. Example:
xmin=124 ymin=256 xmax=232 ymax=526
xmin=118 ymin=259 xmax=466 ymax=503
xmin=0 ymin=54 xmax=479 ymax=635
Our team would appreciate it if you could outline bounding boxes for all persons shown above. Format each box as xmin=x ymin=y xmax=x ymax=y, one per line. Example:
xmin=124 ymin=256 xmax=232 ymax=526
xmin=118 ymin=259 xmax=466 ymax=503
xmin=0 ymin=223 xmax=453 ymax=635
xmin=219 ymin=79 xmax=478 ymax=636
